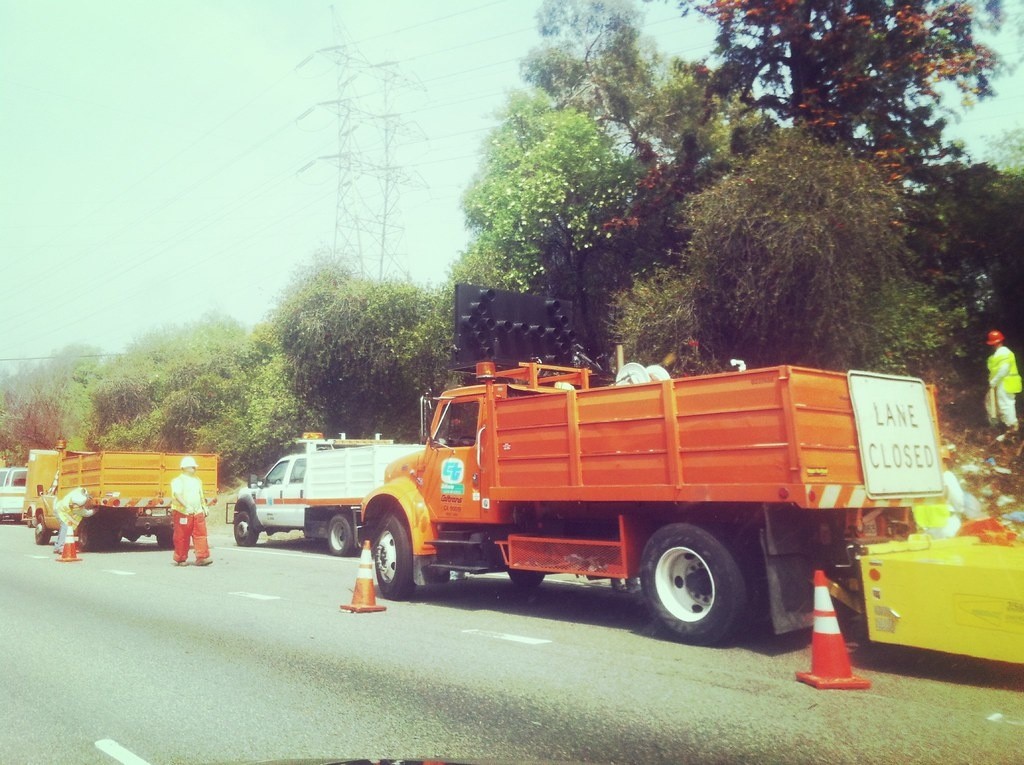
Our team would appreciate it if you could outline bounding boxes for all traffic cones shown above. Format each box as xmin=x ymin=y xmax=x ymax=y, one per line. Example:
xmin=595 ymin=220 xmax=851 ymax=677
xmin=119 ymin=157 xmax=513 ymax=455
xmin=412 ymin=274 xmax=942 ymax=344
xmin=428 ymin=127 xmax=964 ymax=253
xmin=56 ymin=521 xmax=82 ymax=562
xmin=339 ymin=540 xmax=387 ymax=612
xmin=795 ymin=570 xmax=871 ymax=691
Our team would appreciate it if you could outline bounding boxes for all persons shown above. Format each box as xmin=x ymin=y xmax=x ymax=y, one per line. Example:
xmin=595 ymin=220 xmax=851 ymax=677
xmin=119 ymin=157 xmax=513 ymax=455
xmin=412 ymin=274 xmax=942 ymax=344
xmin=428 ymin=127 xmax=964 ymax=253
xmin=171 ymin=456 xmax=213 ymax=567
xmin=983 ymin=329 xmax=1023 ymax=435
xmin=54 ymin=487 xmax=96 ymax=554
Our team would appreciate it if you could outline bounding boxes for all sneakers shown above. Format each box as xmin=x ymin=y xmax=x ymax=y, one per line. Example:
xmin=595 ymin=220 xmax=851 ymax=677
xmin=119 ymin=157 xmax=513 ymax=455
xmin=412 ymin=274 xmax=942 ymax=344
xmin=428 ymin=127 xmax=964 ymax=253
xmin=53 ymin=548 xmax=63 ymax=554
xmin=172 ymin=557 xmax=214 ymax=566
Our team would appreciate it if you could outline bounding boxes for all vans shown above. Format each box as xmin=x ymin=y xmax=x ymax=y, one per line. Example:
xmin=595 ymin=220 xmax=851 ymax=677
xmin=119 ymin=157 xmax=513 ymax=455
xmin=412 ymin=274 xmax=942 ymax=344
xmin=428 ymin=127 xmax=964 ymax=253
xmin=0 ymin=468 xmax=27 ymax=523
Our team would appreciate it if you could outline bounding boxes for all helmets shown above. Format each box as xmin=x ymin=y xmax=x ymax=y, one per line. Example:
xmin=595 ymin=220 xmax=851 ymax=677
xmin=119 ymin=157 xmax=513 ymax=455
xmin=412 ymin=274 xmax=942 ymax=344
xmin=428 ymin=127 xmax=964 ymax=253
xmin=72 ymin=494 xmax=87 ymax=507
xmin=180 ymin=456 xmax=198 ymax=468
xmin=986 ymin=330 xmax=1005 ymax=345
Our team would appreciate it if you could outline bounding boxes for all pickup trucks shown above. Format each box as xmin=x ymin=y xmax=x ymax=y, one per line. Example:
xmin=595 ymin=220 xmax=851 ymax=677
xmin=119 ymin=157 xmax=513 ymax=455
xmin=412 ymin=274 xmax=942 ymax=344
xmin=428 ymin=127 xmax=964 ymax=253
xmin=225 ymin=432 xmax=425 ymax=557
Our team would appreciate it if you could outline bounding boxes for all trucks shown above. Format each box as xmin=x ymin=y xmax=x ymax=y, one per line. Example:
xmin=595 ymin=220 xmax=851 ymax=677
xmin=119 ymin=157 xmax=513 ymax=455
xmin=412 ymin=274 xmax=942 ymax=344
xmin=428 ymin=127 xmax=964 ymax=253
xmin=360 ymin=283 xmax=950 ymax=646
xmin=20 ymin=439 xmax=220 ymax=550
